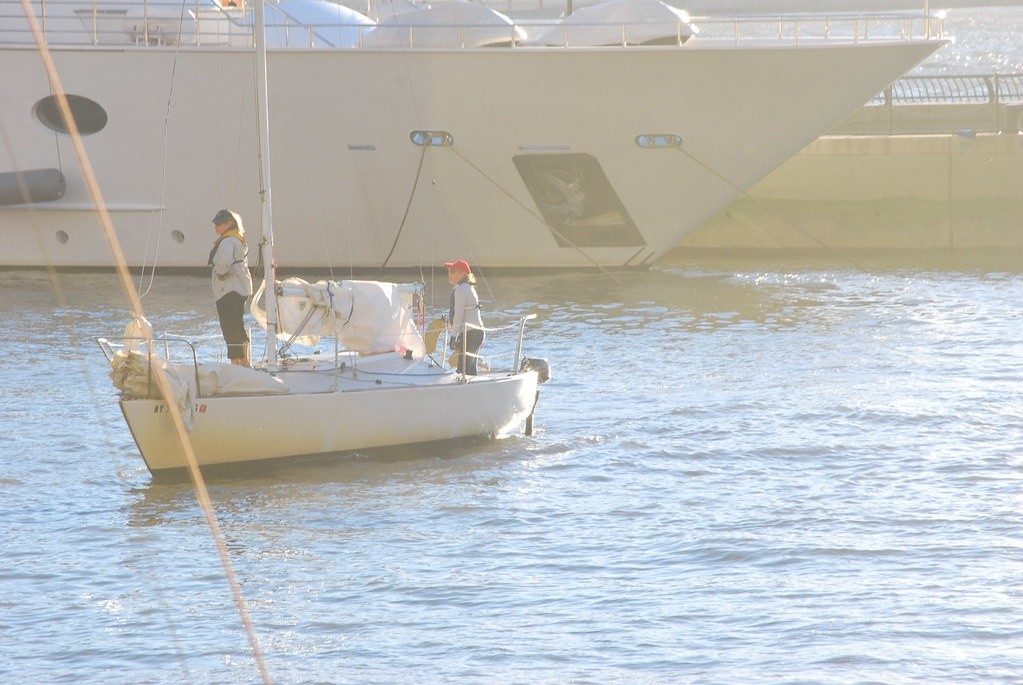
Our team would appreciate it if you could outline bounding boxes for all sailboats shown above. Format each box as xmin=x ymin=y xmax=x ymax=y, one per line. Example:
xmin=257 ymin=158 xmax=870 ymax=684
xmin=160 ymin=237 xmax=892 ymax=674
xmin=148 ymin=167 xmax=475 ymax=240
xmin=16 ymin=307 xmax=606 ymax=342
xmin=96 ymin=1 xmax=550 ymax=482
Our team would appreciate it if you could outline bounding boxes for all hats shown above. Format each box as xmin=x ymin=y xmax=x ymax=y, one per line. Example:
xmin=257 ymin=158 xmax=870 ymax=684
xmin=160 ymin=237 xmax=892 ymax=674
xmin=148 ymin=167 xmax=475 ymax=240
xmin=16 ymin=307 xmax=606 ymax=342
xmin=212 ymin=209 xmax=235 ymax=224
xmin=444 ymin=259 xmax=472 ymax=274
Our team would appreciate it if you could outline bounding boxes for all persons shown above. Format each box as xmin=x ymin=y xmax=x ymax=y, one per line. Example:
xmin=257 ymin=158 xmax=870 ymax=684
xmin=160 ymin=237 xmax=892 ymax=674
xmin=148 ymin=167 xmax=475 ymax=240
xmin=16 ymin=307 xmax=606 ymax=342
xmin=444 ymin=260 xmax=486 ymax=377
xmin=207 ymin=210 xmax=253 ymax=367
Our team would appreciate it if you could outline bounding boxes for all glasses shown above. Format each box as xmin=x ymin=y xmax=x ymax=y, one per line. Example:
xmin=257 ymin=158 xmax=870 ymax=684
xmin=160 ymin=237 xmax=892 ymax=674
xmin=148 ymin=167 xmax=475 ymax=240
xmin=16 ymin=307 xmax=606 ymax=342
xmin=450 ymin=269 xmax=462 ymax=272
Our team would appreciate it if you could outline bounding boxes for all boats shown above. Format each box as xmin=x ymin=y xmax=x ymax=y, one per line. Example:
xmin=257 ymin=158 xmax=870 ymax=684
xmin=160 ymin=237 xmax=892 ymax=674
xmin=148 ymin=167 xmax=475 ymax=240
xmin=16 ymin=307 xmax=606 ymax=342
xmin=357 ymin=5 xmax=528 ymax=48
xmin=537 ymin=0 xmax=699 ymax=47
xmin=1 ymin=0 xmax=957 ymax=276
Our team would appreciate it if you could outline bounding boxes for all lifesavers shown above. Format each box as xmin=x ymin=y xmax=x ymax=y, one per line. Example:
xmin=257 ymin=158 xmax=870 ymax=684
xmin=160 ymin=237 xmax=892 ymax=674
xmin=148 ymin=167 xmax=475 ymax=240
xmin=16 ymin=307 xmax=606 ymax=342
xmin=424 ymin=318 xmax=458 ymax=368
